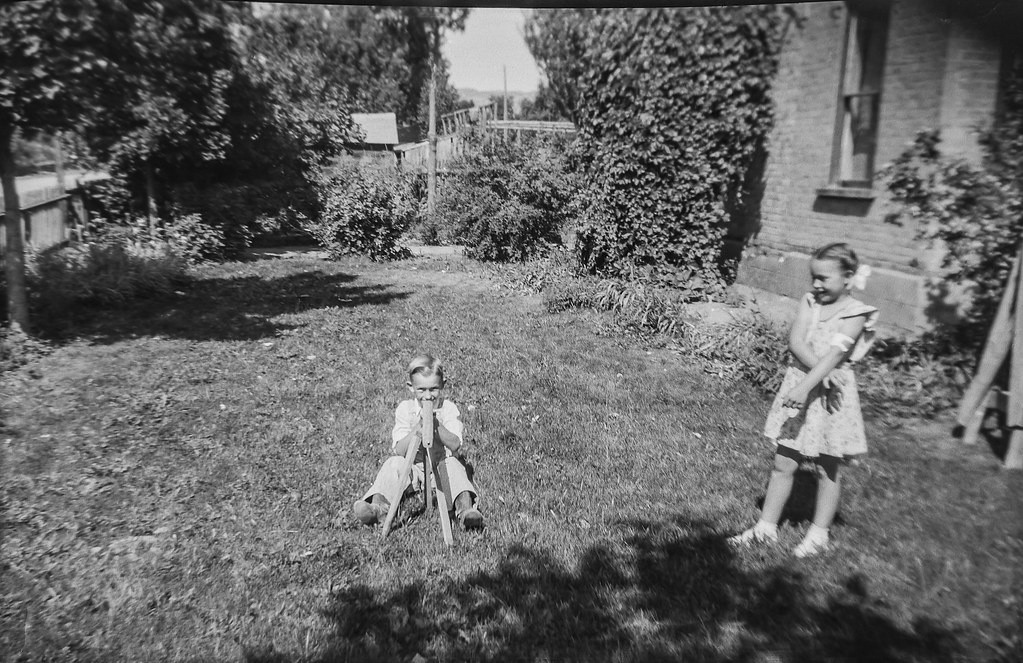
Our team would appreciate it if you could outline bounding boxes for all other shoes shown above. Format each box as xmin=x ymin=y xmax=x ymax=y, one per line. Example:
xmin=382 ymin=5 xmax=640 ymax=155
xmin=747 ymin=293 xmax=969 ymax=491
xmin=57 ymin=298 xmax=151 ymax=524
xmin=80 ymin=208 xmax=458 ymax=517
xmin=790 ymin=536 xmax=831 ymax=557
xmin=456 ymin=508 xmax=487 ymax=531
xmin=354 ymin=498 xmax=393 ymax=524
xmin=726 ymin=528 xmax=777 ymax=546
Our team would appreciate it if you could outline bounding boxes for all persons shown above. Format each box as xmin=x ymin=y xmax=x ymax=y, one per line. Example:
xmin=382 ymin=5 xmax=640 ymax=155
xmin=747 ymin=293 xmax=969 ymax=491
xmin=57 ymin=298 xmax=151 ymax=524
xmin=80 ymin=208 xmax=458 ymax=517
xmin=354 ymin=354 xmax=483 ymax=530
xmin=722 ymin=244 xmax=881 ymax=557
